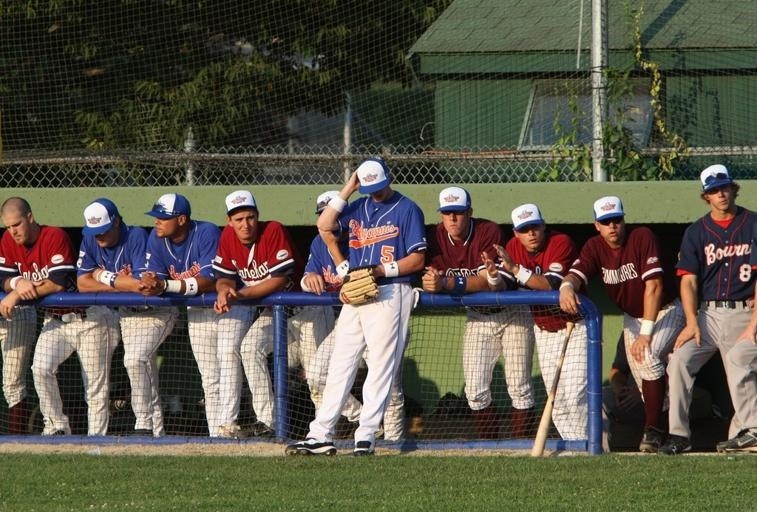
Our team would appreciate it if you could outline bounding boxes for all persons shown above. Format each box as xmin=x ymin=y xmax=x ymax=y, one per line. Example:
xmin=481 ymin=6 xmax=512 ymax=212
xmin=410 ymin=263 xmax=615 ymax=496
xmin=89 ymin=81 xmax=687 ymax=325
xmin=286 ymin=157 xmax=427 ymax=456
xmin=213 ymin=190 xmax=302 ymax=438
xmin=140 ymin=192 xmax=224 ymax=436
xmin=76 ymin=198 xmax=180 ymax=436
xmin=420 ymin=187 xmax=535 ymax=439
xmin=481 ymin=203 xmax=612 ymax=452
xmin=559 ymin=197 xmax=688 ymax=453
xmin=300 ymin=190 xmax=384 ymax=439
xmin=2 ymin=196 xmax=110 ymax=438
xmin=656 ymin=164 xmax=756 ymax=454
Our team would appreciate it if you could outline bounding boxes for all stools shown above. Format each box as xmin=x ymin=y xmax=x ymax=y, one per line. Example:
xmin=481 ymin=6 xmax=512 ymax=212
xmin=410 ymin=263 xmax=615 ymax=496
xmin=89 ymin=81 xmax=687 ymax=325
xmin=707 ymin=301 xmax=746 ymax=310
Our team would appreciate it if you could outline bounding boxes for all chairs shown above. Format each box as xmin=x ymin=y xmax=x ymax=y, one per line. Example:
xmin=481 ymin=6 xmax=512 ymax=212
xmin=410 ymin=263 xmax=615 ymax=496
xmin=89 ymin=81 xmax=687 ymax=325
xmin=231 ymin=420 xmax=274 ymax=437
xmin=286 ymin=438 xmax=336 ymax=456
xmin=353 ymin=441 xmax=375 ymax=455
xmin=638 ymin=422 xmax=693 ymax=455
xmin=716 ymin=432 xmax=757 ymax=453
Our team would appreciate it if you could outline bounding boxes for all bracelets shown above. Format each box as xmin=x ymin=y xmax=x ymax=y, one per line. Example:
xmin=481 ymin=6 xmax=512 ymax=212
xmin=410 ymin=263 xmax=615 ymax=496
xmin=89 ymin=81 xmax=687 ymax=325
xmin=163 ymin=280 xmax=169 ymax=294
xmin=383 ymin=262 xmax=398 ymax=277
xmin=456 ymin=275 xmax=465 ymax=290
xmin=164 ymin=280 xmax=181 ymax=293
xmin=640 ymin=320 xmax=654 ymax=336
xmin=442 ymin=276 xmax=447 ymax=289
xmin=100 ymin=270 xmax=112 ymax=285
xmin=10 ymin=276 xmax=21 ymax=289
xmin=110 ymin=274 xmax=117 ymax=288
xmin=560 ymin=282 xmax=575 ymax=290
xmin=486 ymin=271 xmax=503 ymax=286
xmin=329 ymin=196 xmax=347 ymax=213
xmin=515 ymin=264 xmax=532 ymax=285
xmin=335 ymin=260 xmax=349 ymax=277
xmin=182 ymin=278 xmax=198 ymax=295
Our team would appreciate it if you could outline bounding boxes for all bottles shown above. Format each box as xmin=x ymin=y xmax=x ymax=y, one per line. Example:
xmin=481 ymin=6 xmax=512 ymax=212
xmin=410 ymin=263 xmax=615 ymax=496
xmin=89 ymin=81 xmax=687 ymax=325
xmin=511 ymin=203 xmax=544 ymax=231
xmin=593 ymin=196 xmax=624 ymax=220
xmin=144 ymin=193 xmax=190 ymax=219
xmin=82 ymin=198 xmax=118 ymax=236
xmin=700 ymin=164 xmax=732 ymax=192
xmin=437 ymin=187 xmax=470 ymax=211
xmin=357 ymin=158 xmax=390 ymax=193
xmin=314 ymin=190 xmax=341 ymax=214
xmin=225 ymin=190 xmax=257 ymax=215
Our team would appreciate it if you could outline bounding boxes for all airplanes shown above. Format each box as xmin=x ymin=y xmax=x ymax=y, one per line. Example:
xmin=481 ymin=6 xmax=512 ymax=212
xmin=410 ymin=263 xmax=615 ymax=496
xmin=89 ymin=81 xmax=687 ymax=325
xmin=339 ymin=265 xmax=379 ymax=305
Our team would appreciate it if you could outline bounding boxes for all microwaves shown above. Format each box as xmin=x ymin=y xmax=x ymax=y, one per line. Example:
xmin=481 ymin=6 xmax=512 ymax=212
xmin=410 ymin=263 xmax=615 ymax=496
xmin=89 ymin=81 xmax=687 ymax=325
xmin=531 ymin=322 xmax=575 ymax=458
